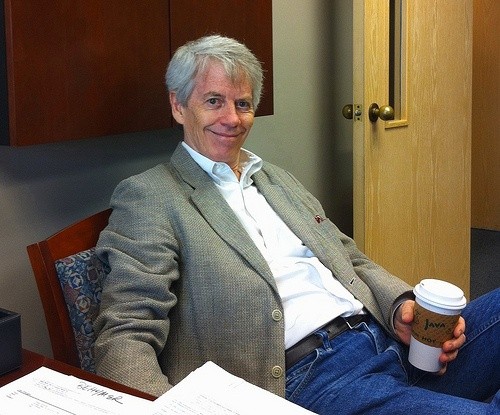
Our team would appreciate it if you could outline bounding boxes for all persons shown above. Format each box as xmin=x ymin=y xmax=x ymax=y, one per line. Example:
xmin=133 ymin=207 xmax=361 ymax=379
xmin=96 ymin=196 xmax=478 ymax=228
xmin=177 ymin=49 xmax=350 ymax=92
xmin=94 ymin=35 xmax=500 ymax=415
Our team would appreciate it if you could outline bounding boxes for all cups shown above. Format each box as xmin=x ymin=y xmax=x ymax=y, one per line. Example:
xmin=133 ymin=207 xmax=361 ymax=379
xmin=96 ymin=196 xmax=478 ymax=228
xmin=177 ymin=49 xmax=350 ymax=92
xmin=407 ymin=278 xmax=467 ymax=372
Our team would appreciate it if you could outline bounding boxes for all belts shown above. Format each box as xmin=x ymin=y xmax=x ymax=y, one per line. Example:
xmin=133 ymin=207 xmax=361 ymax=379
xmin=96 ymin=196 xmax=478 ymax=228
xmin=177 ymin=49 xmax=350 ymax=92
xmin=285 ymin=307 xmax=369 ymax=371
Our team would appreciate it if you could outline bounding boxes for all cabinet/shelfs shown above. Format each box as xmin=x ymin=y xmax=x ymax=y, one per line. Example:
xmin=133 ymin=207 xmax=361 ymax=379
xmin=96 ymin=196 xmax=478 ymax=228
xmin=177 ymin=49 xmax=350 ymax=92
xmin=0 ymin=0 xmax=274 ymax=148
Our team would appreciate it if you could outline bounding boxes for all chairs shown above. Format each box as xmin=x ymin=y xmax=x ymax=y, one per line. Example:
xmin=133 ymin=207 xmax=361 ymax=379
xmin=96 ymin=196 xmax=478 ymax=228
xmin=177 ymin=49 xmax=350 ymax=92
xmin=27 ymin=207 xmax=113 ymax=374
